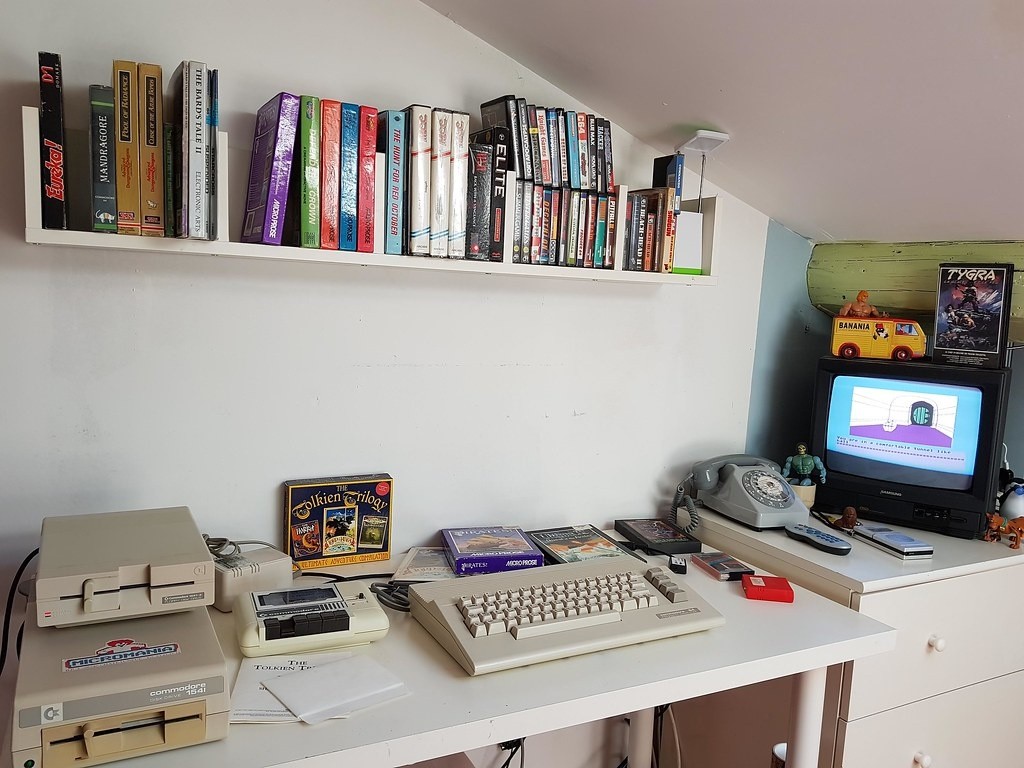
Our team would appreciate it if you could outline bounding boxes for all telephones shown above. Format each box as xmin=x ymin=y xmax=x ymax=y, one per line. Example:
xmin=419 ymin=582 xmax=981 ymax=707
xmin=689 ymin=453 xmax=810 ymax=533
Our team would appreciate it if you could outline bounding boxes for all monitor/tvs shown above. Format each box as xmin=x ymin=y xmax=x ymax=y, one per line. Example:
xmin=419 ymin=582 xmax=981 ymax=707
xmin=807 ymin=356 xmax=1014 ymax=536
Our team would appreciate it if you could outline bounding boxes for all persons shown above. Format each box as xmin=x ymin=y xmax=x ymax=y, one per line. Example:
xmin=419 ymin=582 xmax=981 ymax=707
xmin=840 ymin=290 xmax=890 ymax=317
xmin=834 ymin=507 xmax=863 ymax=530
xmin=782 ymin=442 xmax=826 ymax=486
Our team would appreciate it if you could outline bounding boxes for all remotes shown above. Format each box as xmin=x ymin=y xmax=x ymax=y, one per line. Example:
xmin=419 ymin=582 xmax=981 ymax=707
xmin=784 ymin=522 xmax=852 ymax=557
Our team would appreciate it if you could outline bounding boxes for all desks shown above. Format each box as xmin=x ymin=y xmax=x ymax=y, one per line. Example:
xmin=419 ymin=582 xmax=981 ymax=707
xmin=0 ymin=529 xmax=898 ymax=768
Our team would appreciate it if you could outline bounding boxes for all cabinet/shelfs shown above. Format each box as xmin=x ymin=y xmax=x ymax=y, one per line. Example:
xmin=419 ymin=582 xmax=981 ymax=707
xmin=677 ymin=503 xmax=1024 ymax=768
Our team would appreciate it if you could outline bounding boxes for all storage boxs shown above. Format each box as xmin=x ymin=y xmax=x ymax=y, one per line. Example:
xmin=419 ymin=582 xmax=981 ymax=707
xmin=615 ymin=519 xmax=702 ymax=555
xmin=283 ymin=473 xmax=394 ymax=570
xmin=442 ymin=526 xmax=544 ymax=575
xmin=671 ymin=210 xmax=703 ymax=273
xmin=693 ymin=552 xmax=754 ymax=581
xmin=741 ymin=574 xmax=794 ymax=603
xmin=525 ymin=524 xmax=647 ymax=566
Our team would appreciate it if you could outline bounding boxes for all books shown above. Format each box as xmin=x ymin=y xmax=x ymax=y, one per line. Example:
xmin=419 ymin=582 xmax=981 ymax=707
xmin=240 ymin=95 xmax=685 ymax=274
xmin=38 ymin=51 xmax=229 ymax=242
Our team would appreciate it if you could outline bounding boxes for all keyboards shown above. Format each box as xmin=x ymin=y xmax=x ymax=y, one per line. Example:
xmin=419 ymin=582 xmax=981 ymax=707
xmin=407 ymin=555 xmax=726 ymax=676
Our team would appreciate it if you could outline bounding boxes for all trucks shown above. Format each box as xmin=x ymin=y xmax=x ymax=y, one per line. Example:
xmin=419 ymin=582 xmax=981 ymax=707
xmin=830 ymin=315 xmax=928 ymax=362
